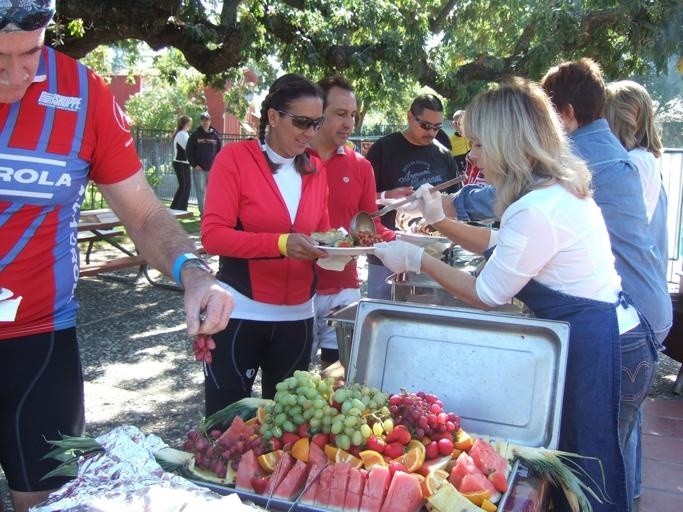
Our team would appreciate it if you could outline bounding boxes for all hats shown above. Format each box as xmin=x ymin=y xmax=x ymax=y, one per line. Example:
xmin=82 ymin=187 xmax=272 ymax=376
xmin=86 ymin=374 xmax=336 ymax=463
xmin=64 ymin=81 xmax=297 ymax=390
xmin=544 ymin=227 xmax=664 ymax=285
xmin=200 ymin=111 xmax=211 ymax=120
xmin=0 ymin=0 xmax=55 ymax=34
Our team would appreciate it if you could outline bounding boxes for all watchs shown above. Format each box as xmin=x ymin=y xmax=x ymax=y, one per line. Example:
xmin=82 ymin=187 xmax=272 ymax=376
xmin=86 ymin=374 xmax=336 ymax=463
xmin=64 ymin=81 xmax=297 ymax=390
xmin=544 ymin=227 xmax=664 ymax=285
xmin=185 ymin=259 xmax=214 ymax=275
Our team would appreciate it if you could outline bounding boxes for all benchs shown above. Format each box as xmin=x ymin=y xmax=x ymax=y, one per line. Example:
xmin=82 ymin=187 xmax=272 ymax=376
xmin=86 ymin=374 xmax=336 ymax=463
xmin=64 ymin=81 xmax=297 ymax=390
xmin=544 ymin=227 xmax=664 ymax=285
xmin=76 ymin=245 xmax=204 ymax=294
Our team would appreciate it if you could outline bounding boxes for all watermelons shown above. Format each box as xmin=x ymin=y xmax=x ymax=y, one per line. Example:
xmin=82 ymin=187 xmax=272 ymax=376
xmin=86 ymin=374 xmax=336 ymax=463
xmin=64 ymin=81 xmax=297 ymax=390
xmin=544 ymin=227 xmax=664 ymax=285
xmin=449 ymin=438 xmax=511 ymax=507
xmin=230 ymin=451 xmax=426 ymax=512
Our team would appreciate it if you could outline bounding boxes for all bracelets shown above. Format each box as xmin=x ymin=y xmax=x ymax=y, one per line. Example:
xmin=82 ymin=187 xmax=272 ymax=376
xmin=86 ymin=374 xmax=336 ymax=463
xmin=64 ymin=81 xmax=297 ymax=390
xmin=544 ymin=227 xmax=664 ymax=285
xmin=171 ymin=252 xmax=200 ymax=290
xmin=277 ymin=233 xmax=291 ymax=256
xmin=380 ymin=189 xmax=387 ymax=201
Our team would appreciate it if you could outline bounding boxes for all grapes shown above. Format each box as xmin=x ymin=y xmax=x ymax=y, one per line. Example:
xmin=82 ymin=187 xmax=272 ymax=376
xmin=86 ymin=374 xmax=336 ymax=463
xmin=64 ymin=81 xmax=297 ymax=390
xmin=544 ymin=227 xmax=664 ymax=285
xmin=260 ymin=370 xmax=394 ymax=450
xmin=185 ymin=422 xmax=246 ymax=480
xmin=366 ymin=385 xmax=464 ymax=458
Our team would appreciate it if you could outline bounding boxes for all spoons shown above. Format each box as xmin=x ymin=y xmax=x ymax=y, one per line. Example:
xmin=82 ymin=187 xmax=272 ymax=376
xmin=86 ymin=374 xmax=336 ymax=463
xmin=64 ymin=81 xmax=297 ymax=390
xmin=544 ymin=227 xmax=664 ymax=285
xmin=349 ymin=175 xmax=466 ymax=240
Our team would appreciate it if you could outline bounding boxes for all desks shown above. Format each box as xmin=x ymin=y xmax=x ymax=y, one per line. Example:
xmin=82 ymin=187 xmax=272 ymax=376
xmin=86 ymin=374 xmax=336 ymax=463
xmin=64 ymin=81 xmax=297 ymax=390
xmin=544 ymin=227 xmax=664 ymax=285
xmin=75 ymin=205 xmax=194 ymax=284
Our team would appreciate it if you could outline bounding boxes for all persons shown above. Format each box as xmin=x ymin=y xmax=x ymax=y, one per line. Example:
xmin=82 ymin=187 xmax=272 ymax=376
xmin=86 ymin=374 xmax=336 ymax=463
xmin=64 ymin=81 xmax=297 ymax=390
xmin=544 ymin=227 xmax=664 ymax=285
xmin=538 ymin=58 xmax=674 ymax=511
xmin=169 ymin=115 xmax=193 ymax=210
xmin=185 ymin=110 xmax=224 ymax=219
xmin=434 ymin=110 xmax=492 ymax=188
xmin=201 ymin=71 xmax=359 ymax=422
xmin=304 ymin=75 xmax=404 ymax=380
xmin=0 ymin=1 xmax=238 ymax=511
xmin=600 ymin=78 xmax=669 ymax=282
xmin=373 ymin=77 xmax=667 ymax=512
xmin=364 ymin=92 xmax=464 ymax=302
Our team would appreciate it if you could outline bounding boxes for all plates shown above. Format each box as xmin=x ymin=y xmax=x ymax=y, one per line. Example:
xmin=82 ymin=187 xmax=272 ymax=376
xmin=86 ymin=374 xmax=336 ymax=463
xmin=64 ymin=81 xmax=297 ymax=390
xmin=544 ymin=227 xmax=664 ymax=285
xmin=396 ymin=230 xmax=448 ymax=240
xmin=315 ymin=245 xmax=376 ymax=256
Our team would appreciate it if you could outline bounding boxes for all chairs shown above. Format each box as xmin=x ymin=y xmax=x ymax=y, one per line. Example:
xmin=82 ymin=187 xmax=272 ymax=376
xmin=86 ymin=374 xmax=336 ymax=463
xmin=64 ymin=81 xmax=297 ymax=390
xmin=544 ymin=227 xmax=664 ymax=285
xmin=75 ymin=230 xmax=125 ymax=279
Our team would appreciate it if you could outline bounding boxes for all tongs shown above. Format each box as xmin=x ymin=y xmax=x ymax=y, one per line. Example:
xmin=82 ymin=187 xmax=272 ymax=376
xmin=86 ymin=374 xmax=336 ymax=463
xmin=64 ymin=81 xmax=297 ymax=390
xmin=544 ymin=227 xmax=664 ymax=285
xmin=264 ymin=453 xmax=332 ymax=511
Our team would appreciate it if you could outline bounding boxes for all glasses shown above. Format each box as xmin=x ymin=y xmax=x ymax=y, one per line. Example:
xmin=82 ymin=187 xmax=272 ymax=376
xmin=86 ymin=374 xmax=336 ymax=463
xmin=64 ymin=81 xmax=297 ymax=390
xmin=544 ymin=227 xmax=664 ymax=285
xmin=408 ymin=108 xmax=443 ymax=130
xmin=0 ymin=7 xmax=54 ymax=31
xmin=277 ymin=109 xmax=325 ymax=131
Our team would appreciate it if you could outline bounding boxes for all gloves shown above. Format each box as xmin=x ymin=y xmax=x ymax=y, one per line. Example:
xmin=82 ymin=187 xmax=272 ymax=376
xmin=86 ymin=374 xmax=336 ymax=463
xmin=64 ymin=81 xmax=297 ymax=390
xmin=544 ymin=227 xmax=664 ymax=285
xmin=409 ymin=182 xmax=446 ymax=227
xmin=372 ymin=239 xmax=425 ymax=275
